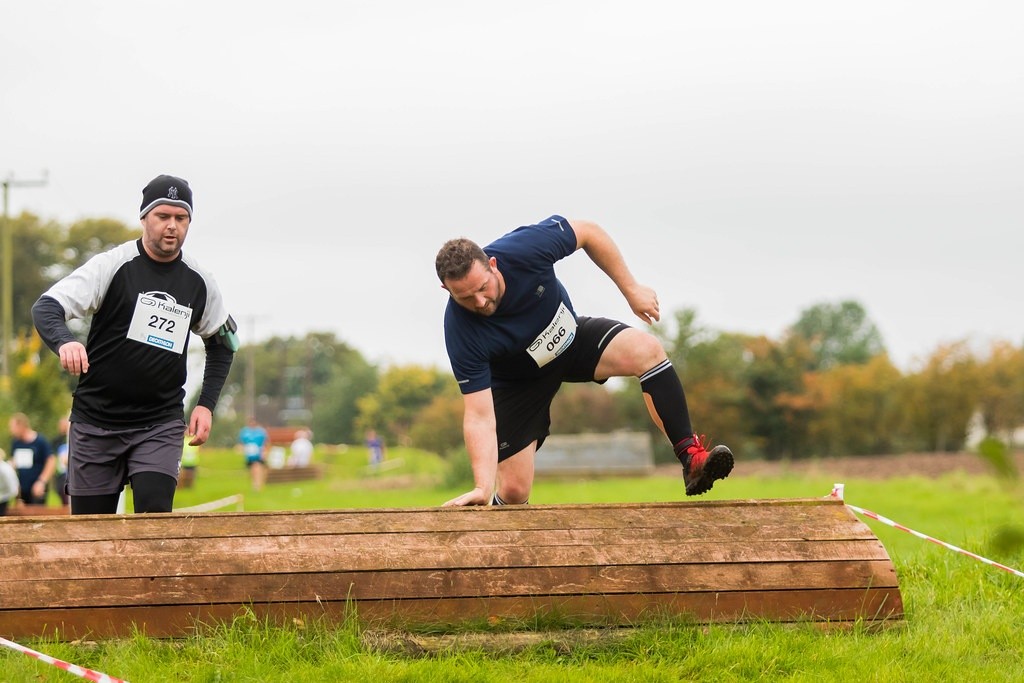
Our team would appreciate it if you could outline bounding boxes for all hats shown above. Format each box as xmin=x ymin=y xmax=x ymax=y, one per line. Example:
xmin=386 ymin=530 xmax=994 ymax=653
xmin=139 ymin=174 xmax=193 ymax=223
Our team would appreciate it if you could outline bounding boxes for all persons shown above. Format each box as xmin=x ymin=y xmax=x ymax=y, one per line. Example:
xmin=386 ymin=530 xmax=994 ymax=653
xmin=49 ymin=416 xmax=70 ymax=508
xmin=6 ymin=411 xmax=56 ymax=506
xmin=29 ymin=173 xmax=240 ymax=517
xmin=181 ymin=422 xmax=386 ymax=490
xmin=433 ymin=212 xmax=735 ymax=510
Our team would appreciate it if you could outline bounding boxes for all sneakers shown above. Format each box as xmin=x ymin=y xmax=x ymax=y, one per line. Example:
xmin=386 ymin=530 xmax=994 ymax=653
xmin=682 ymin=431 xmax=734 ymax=496
